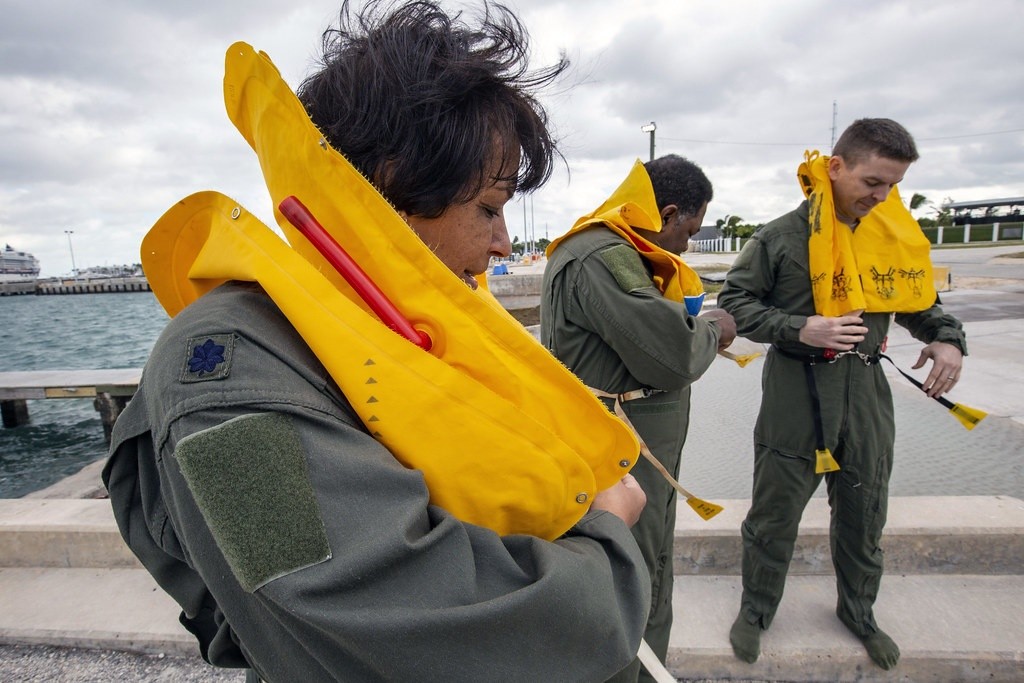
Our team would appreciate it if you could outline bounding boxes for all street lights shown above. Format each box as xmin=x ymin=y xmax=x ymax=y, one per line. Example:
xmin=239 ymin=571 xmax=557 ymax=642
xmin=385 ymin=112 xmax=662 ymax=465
xmin=65 ymin=229 xmax=78 ymax=289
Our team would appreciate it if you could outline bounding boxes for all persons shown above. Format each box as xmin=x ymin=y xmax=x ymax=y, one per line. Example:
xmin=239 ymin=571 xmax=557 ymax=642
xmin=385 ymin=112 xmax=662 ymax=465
xmin=538 ymin=153 xmax=736 ymax=683
xmin=717 ymin=119 xmax=968 ymax=671
xmin=102 ymin=3 xmax=650 ymax=683
xmin=492 ymin=253 xmax=520 ymax=264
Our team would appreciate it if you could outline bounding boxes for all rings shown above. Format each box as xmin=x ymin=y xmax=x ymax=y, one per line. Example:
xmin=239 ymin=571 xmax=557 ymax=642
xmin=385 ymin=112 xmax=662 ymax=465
xmin=948 ymin=377 xmax=954 ymax=380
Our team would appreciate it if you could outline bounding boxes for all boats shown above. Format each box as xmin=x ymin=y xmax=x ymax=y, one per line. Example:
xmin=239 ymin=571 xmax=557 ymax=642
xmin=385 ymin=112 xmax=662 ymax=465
xmin=0 ymin=244 xmax=42 ymax=295
